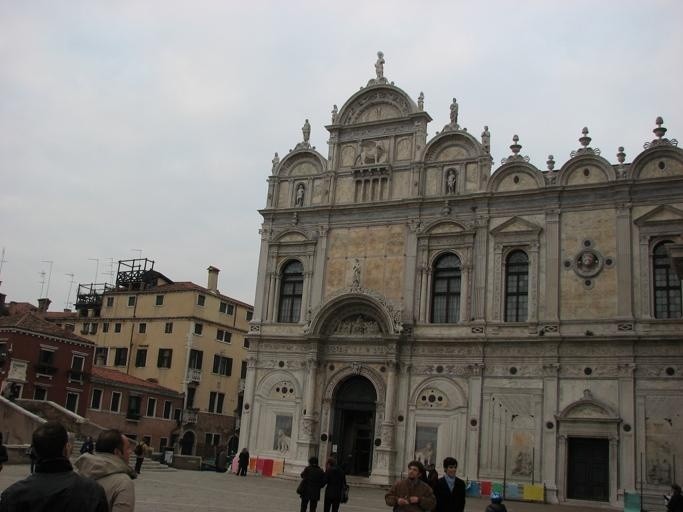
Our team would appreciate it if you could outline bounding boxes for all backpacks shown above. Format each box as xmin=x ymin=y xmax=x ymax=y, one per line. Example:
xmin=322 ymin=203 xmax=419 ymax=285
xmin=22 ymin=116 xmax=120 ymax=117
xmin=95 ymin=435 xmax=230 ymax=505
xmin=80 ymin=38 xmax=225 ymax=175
xmin=134 ymin=443 xmax=144 ymax=456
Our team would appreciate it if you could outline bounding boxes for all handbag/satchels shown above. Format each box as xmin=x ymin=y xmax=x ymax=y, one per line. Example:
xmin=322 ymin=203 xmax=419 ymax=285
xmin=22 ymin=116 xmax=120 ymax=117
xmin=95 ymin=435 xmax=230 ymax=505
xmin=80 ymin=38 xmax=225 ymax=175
xmin=341 ymin=484 xmax=350 ymax=503
xmin=297 ymin=478 xmax=313 ymax=494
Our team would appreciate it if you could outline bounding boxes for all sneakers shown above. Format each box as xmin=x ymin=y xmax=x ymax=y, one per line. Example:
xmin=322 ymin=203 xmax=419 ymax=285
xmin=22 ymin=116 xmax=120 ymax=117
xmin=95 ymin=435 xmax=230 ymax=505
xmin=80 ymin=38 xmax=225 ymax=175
xmin=236 ymin=473 xmax=247 ymax=476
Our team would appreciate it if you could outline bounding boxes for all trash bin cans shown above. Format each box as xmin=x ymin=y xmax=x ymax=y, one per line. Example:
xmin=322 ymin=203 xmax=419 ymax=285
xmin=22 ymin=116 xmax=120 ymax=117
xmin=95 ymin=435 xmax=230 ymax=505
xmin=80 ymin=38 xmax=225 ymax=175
xmin=624 ymin=490 xmax=641 ymax=512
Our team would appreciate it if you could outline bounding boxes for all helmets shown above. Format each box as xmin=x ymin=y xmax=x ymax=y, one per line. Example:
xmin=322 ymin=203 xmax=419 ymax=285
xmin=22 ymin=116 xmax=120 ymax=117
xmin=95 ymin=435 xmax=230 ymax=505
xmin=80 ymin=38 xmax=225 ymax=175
xmin=490 ymin=491 xmax=504 ymax=500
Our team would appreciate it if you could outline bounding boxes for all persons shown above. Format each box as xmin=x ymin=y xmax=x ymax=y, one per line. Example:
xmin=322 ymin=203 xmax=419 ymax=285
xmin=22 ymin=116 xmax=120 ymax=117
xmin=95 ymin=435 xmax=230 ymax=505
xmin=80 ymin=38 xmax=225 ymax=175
xmin=295 ymin=184 xmax=304 ymax=205
xmin=666 ymin=482 xmax=682 ymax=512
xmin=6 ymin=381 xmax=19 ymax=404
xmin=22 ymin=440 xmax=36 ymax=474
xmin=243 ymin=448 xmax=249 ymax=476
xmin=319 ymin=457 xmax=347 ymax=512
xmin=426 ymin=461 xmax=438 ymax=484
xmin=329 ymin=104 xmax=338 ymax=124
xmin=301 ymin=117 xmax=310 ymax=144
xmin=384 ymin=459 xmax=437 ymax=511
xmin=134 ymin=435 xmax=150 ymax=476
xmin=271 ymin=152 xmax=279 ymax=174
xmin=79 ymin=434 xmax=94 ymax=455
xmin=483 ymin=490 xmax=508 ymax=511
xmin=72 ymin=428 xmax=135 ymax=511
xmin=234 ymin=447 xmax=245 ymax=476
xmin=373 ymin=51 xmax=385 ymax=80
xmin=416 ymin=92 xmax=425 ymax=112
xmin=448 ymin=97 xmax=459 ymax=127
xmin=0 ymin=432 xmax=8 ymax=473
xmin=433 ymin=457 xmax=465 ymax=511
xmin=480 ymin=125 xmax=490 ymax=151
xmin=0 ymin=419 xmax=110 ymax=511
xmin=446 ymin=170 xmax=456 ymax=193
xmin=295 ymin=456 xmax=323 ymax=511
